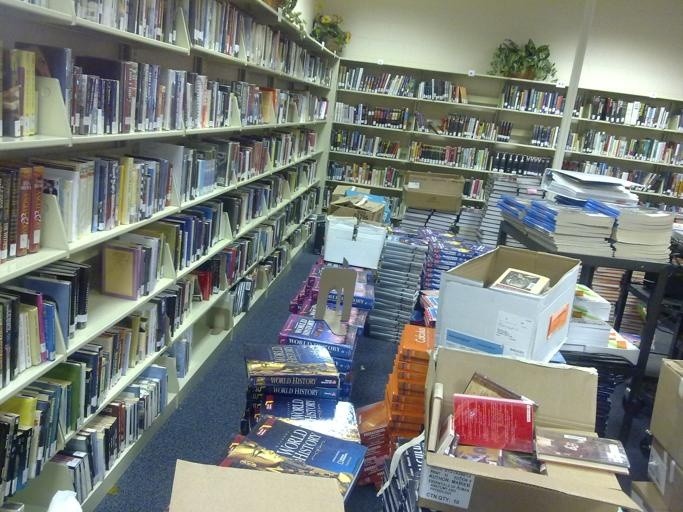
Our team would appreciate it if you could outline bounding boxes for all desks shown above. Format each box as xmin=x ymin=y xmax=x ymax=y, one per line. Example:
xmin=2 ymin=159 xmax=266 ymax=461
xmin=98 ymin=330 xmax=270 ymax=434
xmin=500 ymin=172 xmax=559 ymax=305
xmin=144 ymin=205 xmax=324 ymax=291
xmin=495 ymin=222 xmax=683 ymax=441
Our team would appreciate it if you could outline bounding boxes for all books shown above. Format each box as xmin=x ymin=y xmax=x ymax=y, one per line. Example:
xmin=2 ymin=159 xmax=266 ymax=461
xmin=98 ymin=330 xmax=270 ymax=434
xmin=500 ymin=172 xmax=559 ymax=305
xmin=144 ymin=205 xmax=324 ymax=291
xmin=2 ymin=1 xmax=682 ymax=512
xmin=356 ymin=126 xmax=361 ymax=129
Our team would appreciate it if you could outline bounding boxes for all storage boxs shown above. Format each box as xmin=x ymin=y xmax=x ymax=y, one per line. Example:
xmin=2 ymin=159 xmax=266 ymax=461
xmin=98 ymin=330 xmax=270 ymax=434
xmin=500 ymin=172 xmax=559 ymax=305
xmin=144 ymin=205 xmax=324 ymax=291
xmin=436 ymin=245 xmax=583 ymax=362
xmin=332 ymin=184 xmax=371 ymax=202
xmin=416 ymin=430 xmax=642 ymax=511
xmin=330 ymin=198 xmax=385 ymax=222
xmin=424 ymin=347 xmax=599 ymax=441
xmin=646 ymin=438 xmax=683 ymax=511
xmin=402 ymin=170 xmax=465 ymax=214
xmin=649 ymin=358 xmax=683 ymax=469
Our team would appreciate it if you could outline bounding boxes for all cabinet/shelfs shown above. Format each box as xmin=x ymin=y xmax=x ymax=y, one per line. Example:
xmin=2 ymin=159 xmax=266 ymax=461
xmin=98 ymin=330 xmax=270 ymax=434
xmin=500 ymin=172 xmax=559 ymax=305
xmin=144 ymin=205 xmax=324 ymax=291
xmin=318 ymin=57 xmax=683 ymax=215
xmin=0 ymin=1 xmax=342 ymax=511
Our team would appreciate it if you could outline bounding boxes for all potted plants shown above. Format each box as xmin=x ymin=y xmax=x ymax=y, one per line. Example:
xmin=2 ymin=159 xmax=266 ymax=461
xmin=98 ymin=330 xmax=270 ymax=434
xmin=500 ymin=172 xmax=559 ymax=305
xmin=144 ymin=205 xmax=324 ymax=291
xmin=489 ymin=38 xmax=558 ymax=82
xmin=263 ymin=0 xmax=307 ymax=40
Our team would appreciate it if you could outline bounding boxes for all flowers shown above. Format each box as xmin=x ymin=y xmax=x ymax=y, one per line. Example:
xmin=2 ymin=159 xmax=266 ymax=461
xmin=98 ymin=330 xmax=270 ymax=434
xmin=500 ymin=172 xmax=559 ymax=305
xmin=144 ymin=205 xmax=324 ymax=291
xmin=309 ymin=13 xmax=352 ymax=43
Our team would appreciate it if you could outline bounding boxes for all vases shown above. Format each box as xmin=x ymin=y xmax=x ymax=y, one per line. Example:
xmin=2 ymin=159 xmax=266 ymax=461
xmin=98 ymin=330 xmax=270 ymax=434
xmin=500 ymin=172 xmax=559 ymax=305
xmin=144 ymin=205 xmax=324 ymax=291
xmin=322 ymin=36 xmax=339 ymax=52
